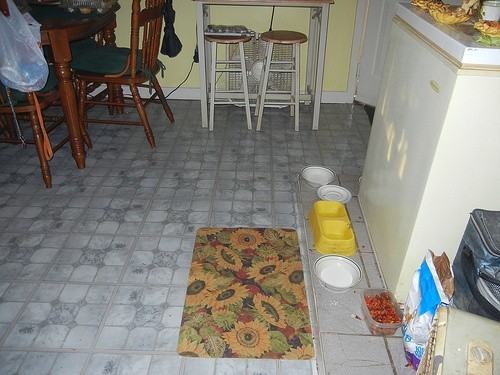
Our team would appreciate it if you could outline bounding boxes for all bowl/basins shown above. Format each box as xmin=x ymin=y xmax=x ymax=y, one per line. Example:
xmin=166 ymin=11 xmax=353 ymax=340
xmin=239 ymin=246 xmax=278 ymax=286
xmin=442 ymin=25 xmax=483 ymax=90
xmin=301 ymin=165 xmax=336 ymax=189
xmin=359 ymin=287 xmax=405 ymax=336
xmin=310 ymin=201 xmax=357 ymax=256
xmin=312 ymin=254 xmax=363 ymax=294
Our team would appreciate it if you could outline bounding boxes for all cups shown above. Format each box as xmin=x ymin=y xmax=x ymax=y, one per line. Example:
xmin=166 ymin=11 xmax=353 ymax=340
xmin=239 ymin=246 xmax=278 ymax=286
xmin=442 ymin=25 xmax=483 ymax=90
xmin=480 ymin=1 xmax=500 ymax=22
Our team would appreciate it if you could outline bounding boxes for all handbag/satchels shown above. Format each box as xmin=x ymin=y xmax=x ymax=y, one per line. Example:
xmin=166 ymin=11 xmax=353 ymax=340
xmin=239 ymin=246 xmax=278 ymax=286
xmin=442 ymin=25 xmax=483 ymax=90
xmin=452 ymin=209 xmax=500 ymax=323
xmin=401 ymin=248 xmax=455 ymax=371
xmin=0 ymin=0 xmax=48 ymax=92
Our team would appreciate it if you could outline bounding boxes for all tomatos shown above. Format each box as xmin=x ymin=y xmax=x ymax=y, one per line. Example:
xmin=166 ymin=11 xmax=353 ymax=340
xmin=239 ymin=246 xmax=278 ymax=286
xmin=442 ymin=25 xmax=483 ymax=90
xmin=365 ymin=292 xmax=401 ymax=332
xmin=483 ymin=21 xmax=500 ymax=29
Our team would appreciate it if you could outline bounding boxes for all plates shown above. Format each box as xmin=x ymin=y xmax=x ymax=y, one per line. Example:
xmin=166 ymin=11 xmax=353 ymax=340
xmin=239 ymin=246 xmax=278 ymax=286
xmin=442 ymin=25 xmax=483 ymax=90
xmin=317 ymin=185 xmax=353 ymax=204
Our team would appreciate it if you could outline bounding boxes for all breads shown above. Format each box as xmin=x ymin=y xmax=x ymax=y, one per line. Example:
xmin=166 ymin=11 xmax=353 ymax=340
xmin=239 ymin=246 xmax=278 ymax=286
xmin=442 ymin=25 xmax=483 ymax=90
xmin=79 ymin=7 xmax=92 ymax=14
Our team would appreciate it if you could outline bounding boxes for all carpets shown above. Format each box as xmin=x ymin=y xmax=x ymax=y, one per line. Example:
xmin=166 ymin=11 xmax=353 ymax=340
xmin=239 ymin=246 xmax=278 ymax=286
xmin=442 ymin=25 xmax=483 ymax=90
xmin=178 ymin=226 xmax=317 ymax=360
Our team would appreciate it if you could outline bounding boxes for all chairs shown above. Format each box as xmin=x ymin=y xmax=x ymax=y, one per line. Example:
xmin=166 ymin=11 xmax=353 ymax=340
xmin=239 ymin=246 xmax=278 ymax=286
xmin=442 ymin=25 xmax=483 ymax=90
xmin=0 ymin=1 xmax=176 ymax=187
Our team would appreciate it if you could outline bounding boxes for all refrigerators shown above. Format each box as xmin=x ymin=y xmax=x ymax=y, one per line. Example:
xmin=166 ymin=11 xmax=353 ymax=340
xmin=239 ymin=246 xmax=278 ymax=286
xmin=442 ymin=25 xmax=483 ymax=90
xmin=357 ymin=2 xmax=500 ymax=302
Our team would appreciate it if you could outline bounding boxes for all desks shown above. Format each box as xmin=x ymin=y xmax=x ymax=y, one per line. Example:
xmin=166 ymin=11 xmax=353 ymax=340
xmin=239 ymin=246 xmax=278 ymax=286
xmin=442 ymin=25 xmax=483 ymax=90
xmin=195 ymin=0 xmax=336 ymax=127
xmin=0 ymin=0 xmax=122 ymax=169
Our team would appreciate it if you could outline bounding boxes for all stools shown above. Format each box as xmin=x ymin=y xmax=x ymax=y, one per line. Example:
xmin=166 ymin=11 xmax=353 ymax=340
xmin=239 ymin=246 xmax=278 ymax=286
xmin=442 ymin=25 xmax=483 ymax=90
xmin=254 ymin=31 xmax=308 ymax=131
xmin=202 ymin=27 xmax=253 ymax=129
